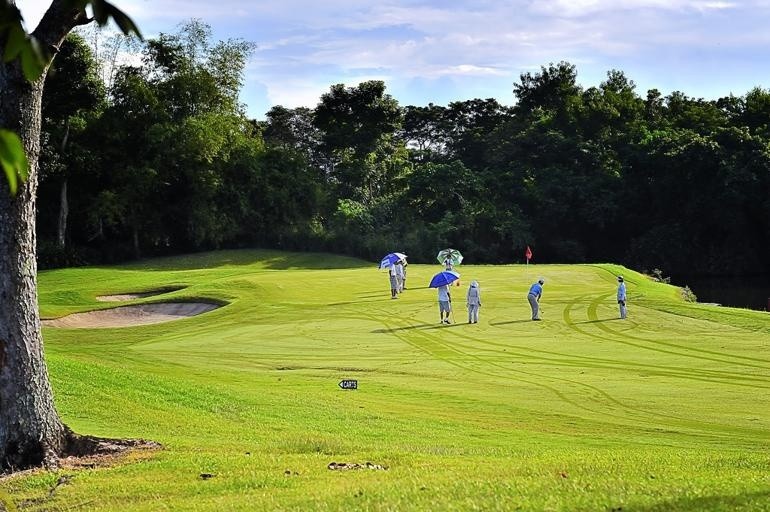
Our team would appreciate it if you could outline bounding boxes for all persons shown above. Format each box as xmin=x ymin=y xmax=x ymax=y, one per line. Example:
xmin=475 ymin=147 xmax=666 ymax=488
xmin=389 ymin=260 xmax=399 ymax=298
xmin=401 ymin=258 xmax=408 ymax=289
xmin=466 ymin=281 xmax=482 ymax=324
xmin=528 ymin=279 xmax=544 ymax=320
xmin=618 ymin=275 xmax=627 ymax=319
xmin=396 ymin=260 xmax=404 ymax=292
xmin=437 ymin=284 xmax=451 ymax=324
xmin=444 ymin=255 xmax=453 ymax=285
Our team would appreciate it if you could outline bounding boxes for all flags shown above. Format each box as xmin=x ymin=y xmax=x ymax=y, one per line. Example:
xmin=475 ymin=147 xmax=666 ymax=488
xmin=525 ymin=247 xmax=532 ymax=259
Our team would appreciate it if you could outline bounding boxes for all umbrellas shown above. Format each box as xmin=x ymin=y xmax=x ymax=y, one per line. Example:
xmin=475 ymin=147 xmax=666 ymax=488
xmin=379 ymin=252 xmax=409 ymax=269
xmin=437 ymin=248 xmax=464 ymax=266
xmin=429 ymin=271 xmax=461 ymax=289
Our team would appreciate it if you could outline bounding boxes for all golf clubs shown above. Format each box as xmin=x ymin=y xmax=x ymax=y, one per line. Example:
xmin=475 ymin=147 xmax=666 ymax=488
xmin=450 ymin=302 xmax=457 ymax=323
xmin=538 ymin=303 xmax=544 ymax=313
